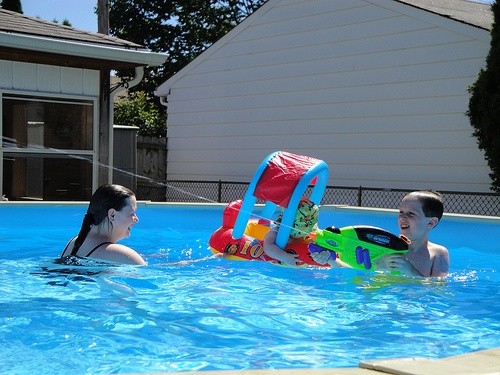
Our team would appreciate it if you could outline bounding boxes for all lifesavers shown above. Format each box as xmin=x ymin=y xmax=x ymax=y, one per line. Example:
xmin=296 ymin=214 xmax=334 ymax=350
xmin=207 ymin=150 xmax=334 ymax=266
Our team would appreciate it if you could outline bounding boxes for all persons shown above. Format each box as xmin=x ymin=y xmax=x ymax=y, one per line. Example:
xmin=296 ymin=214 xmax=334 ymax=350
xmin=61 ymin=184 xmax=146 ymax=265
xmin=263 ymin=191 xmax=448 ymax=280
